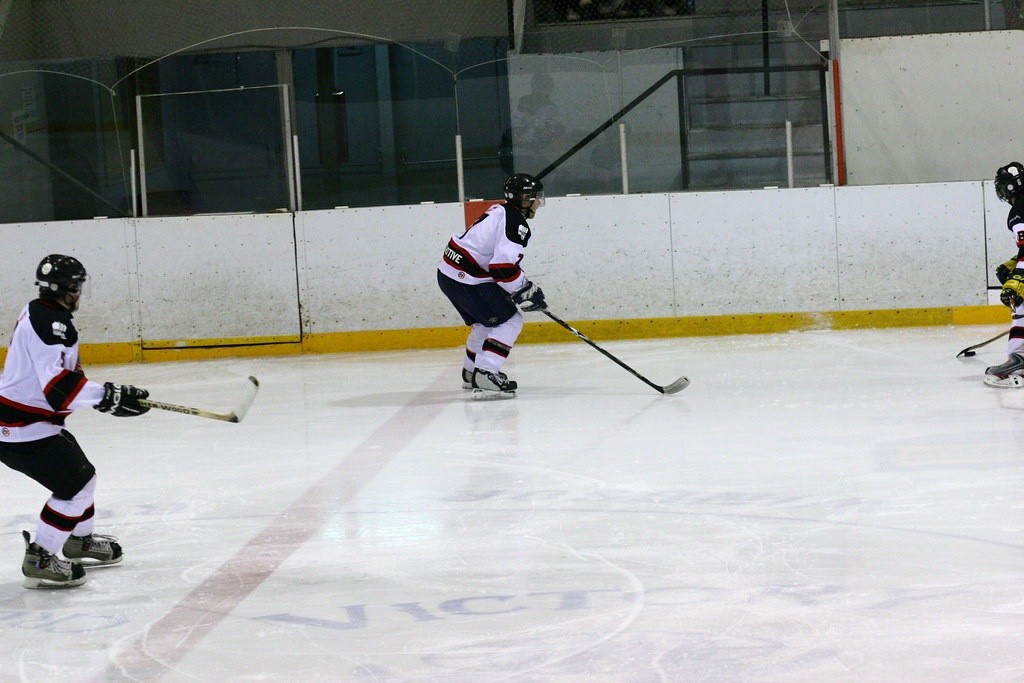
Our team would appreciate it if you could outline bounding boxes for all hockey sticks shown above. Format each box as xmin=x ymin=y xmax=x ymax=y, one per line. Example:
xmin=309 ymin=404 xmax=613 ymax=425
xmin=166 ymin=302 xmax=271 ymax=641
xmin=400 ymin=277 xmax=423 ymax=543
xmin=956 ymin=329 xmax=1010 ymax=357
xmin=542 ymin=307 xmax=690 ymax=394
xmin=134 ymin=375 xmax=259 ymax=422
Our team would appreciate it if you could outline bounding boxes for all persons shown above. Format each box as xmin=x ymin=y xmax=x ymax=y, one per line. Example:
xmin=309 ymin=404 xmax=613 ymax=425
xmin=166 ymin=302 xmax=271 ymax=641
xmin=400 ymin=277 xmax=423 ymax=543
xmin=437 ymin=173 xmax=549 ymax=400
xmin=0 ymin=254 xmax=151 ymax=590
xmin=982 ymin=162 xmax=1024 ymax=388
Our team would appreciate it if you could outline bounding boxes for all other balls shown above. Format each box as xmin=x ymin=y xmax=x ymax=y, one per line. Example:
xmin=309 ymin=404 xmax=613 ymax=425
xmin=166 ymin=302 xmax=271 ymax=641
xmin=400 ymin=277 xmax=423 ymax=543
xmin=965 ymin=351 xmax=976 ymax=357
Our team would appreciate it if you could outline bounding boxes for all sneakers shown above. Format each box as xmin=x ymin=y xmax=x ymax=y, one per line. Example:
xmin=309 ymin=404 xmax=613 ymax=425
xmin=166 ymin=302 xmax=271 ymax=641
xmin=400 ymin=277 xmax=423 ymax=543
xmin=461 ymin=368 xmax=507 ymax=389
xmin=472 ymin=368 xmax=517 ymax=400
xmin=63 ymin=533 xmax=123 ymax=568
xmin=22 ymin=530 xmax=88 ymax=589
xmin=984 ymin=353 xmax=1024 ymax=388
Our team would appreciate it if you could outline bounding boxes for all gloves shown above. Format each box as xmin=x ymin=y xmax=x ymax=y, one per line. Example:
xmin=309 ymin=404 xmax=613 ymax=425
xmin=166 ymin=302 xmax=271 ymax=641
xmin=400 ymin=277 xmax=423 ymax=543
xmin=996 ymin=255 xmax=1018 ymax=284
xmin=93 ymin=382 xmax=149 ymax=418
xmin=1000 ymin=268 xmax=1024 ymax=307
xmin=511 ymin=281 xmax=547 ymax=312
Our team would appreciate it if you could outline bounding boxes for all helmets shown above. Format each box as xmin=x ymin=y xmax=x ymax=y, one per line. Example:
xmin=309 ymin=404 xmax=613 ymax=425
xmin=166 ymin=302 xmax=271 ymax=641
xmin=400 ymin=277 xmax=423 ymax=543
xmin=34 ymin=254 xmax=88 ymax=311
xmin=994 ymin=162 xmax=1024 ymax=198
xmin=503 ymin=173 xmax=543 ymax=219
